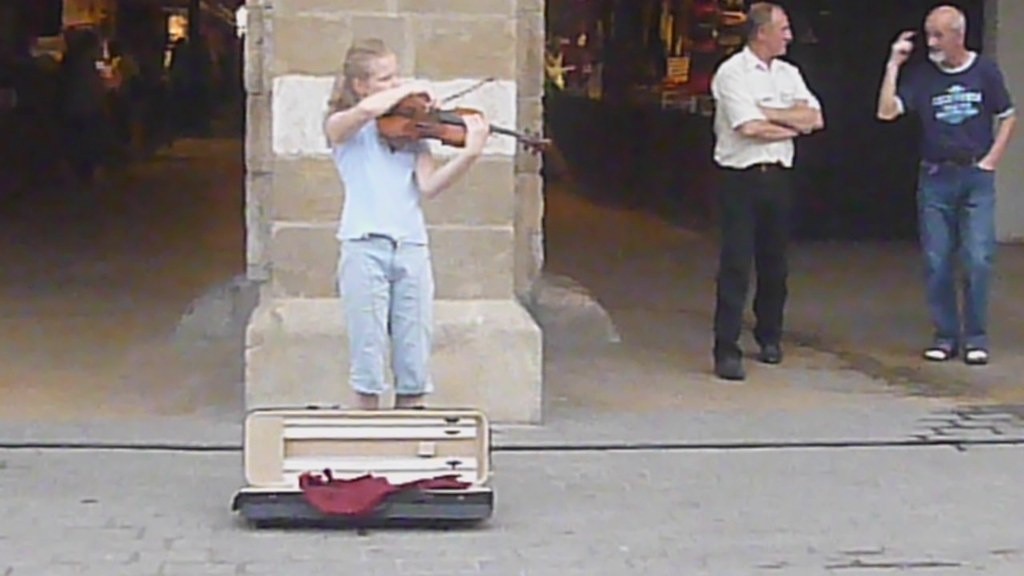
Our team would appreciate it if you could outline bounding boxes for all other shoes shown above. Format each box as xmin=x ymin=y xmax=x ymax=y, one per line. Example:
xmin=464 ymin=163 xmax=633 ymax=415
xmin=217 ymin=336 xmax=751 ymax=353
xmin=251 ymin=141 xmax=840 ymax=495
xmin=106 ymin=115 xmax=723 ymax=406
xmin=714 ymin=350 xmax=745 ymax=379
xmin=761 ymin=342 xmax=782 ymax=362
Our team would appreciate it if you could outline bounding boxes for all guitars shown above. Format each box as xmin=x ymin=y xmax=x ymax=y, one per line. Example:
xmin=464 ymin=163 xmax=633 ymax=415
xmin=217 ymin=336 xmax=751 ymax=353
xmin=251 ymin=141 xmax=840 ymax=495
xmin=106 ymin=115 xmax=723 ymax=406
xmin=375 ymin=91 xmax=553 ymax=156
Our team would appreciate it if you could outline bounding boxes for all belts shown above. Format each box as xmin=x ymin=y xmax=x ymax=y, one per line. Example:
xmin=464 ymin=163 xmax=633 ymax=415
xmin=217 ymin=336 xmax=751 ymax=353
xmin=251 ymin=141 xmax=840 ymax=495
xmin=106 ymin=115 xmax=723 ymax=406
xmin=749 ymin=164 xmax=783 ymax=172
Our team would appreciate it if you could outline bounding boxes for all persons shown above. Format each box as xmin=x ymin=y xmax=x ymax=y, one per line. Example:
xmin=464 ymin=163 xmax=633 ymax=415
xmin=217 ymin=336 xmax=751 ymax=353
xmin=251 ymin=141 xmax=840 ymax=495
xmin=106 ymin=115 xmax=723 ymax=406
xmin=708 ymin=4 xmax=824 ymax=381
xmin=877 ymin=5 xmax=1018 ymax=364
xmin=321 ymin=37 xmax=489 ymax=409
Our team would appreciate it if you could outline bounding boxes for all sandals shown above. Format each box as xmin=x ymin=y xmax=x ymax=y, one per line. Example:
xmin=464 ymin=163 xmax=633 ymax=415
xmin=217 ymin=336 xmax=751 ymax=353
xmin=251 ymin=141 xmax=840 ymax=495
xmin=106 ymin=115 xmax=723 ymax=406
xmin=923 ymin=346 xmax=960 ymax=360
xmin=963 ymin=341 xmax=989 ymax=364
